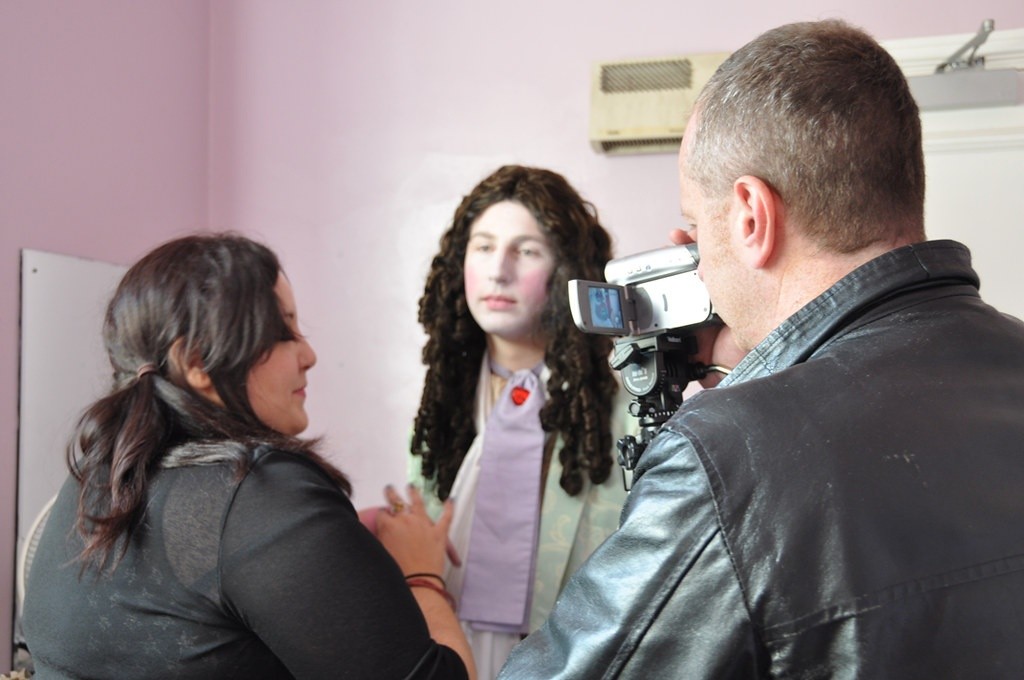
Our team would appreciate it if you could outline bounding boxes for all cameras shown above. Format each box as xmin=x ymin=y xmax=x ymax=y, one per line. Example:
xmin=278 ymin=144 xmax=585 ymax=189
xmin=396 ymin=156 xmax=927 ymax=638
xmin=567 ymin=243 xmax=721 ymax=337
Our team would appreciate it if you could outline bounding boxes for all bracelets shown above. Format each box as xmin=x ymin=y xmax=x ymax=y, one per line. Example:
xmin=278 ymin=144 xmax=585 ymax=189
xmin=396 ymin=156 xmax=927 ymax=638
xmin=405 ymin=573 xmax=446 ymax=589
xmin=407 ymin=578 xmax=456 ymax=611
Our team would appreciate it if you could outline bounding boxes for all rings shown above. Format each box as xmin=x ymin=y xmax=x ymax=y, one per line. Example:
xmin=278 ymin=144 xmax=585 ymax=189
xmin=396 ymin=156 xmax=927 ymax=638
xmin=392 ymin=502 xmax=406 ymax=512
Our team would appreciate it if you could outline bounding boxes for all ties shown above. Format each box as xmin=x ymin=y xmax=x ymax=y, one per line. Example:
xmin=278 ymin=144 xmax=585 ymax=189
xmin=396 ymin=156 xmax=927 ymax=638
xmin=456 ymin=352 xmax=548 ymax=636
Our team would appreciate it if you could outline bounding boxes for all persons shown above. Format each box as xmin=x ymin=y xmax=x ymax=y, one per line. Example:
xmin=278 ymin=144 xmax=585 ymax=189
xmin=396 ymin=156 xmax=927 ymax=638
xmin=411 ymin=165 xmax=659 ymax=680
xmin=499 ymin=20 xmax=1024 ymax=680
xmin=16 ymin=231 xmax=477 ymax=680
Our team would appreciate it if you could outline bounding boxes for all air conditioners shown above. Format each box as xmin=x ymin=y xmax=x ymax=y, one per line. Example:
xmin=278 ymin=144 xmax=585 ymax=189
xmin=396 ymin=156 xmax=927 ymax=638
xmin=585 ymin=54 xmax=733 ymax=156
